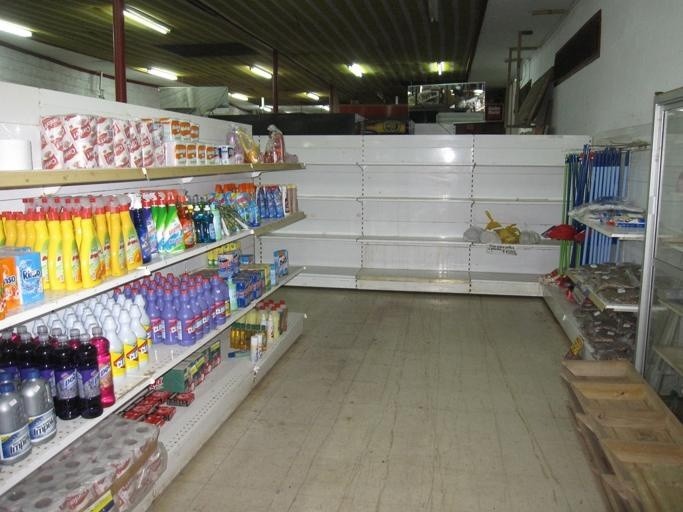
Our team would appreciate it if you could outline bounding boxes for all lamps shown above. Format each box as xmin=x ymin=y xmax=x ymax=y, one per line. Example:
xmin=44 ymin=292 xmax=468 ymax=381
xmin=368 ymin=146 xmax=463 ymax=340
xmin=346 ymin=64 xmax=363 ymax=79
xmin=146 ymin=66 xmax=176 ymax=81
xmin=121 ymin=8 xmax=171 ymax=35
xmin=250 ymin=65 xmax=272 ymax=80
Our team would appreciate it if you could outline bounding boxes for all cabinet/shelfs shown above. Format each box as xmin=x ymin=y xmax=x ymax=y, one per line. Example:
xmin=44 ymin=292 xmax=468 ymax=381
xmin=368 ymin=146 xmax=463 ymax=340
xmin=542 ymin=142 xmax=678 ymax=364
xmin=633 ymin=86 xmax=683 ymax=420
xmin=251 ymin=133 xmax=593 ymax=298
xmin=0 ymin=81 xmax=307 ymax=512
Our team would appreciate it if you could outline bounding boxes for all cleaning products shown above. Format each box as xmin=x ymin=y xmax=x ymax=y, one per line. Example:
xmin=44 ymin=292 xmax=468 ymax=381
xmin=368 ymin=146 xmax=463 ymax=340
xmin=228 ymin=301 xmax=289 ymax=361
xmin=1 ymin=189 xmax=230 ymax=463
xmin=213 ymin=181 xmax=299 ymax=230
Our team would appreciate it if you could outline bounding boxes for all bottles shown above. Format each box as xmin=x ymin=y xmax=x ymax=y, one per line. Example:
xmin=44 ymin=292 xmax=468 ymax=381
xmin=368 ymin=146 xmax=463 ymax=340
xmin=365 ymin=119 xmax=409 ymax=136
xmin=1 ymin=272 xmax=287 ymax=467
